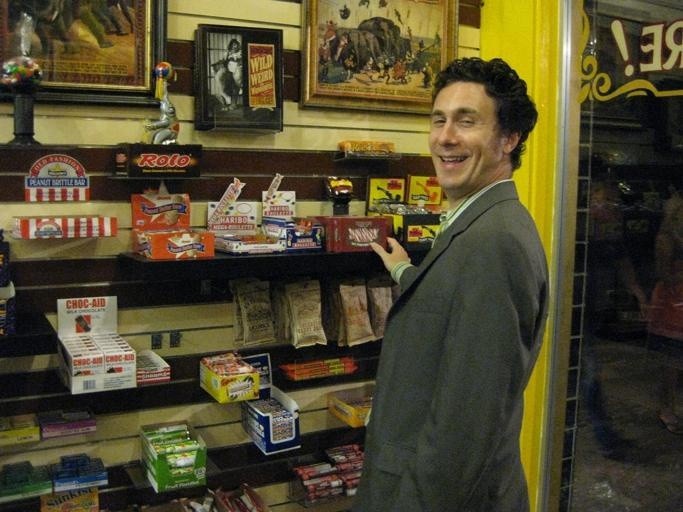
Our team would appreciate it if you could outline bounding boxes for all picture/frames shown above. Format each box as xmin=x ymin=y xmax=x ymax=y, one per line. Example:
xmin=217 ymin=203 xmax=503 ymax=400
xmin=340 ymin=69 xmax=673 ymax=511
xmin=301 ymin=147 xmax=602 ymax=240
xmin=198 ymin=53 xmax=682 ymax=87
xmin=299 ymin=0 xmax=460 ymax=117
xmin=0 ymin=1 xmax=169 ymax=108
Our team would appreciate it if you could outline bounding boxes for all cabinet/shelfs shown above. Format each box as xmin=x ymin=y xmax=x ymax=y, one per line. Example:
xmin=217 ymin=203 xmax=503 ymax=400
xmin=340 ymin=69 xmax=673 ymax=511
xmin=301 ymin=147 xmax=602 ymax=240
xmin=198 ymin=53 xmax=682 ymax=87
xmin=0 ymin=239 xmax=430 ymax=512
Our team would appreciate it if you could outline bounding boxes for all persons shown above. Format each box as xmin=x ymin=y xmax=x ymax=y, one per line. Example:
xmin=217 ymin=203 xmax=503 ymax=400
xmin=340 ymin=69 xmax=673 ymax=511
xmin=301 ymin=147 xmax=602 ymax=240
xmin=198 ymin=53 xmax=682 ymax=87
xmin=319 ymin=20 xmax=433 ymax=88
xmin=567 ymin=180 xmax=646 ymax=467
xmin=353 ymin=57 xmax=550 ymax=512
xmin=220 ymin=39 xmax=242 ymax=105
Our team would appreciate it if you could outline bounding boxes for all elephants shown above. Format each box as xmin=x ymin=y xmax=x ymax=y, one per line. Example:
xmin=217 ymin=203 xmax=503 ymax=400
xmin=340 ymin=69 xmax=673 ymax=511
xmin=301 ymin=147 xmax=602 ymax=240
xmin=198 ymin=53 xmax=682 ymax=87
xmin=6 ymin=0 xmax=135 ymax=59
xmin=320 ymin=17 xmax=411 ymax=83
